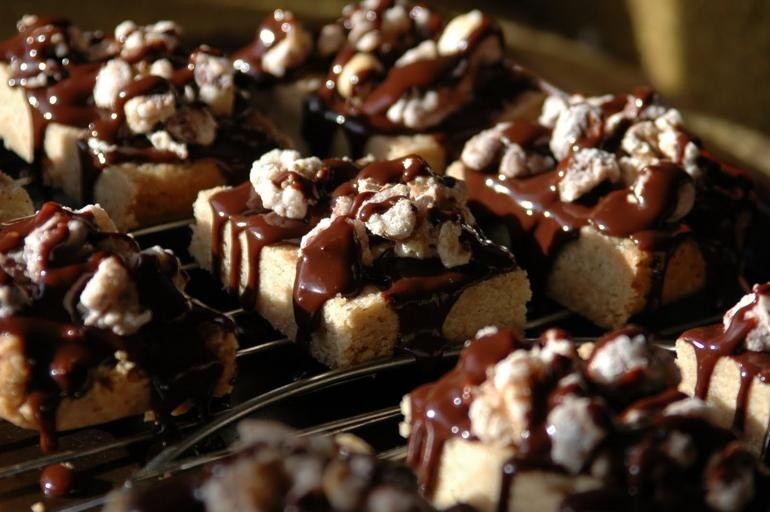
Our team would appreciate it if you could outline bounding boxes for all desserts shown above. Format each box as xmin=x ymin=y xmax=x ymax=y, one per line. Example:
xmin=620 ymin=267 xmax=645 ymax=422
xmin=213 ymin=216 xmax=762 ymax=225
xmin=0 ymin=0 xmax=770 ymax=512
